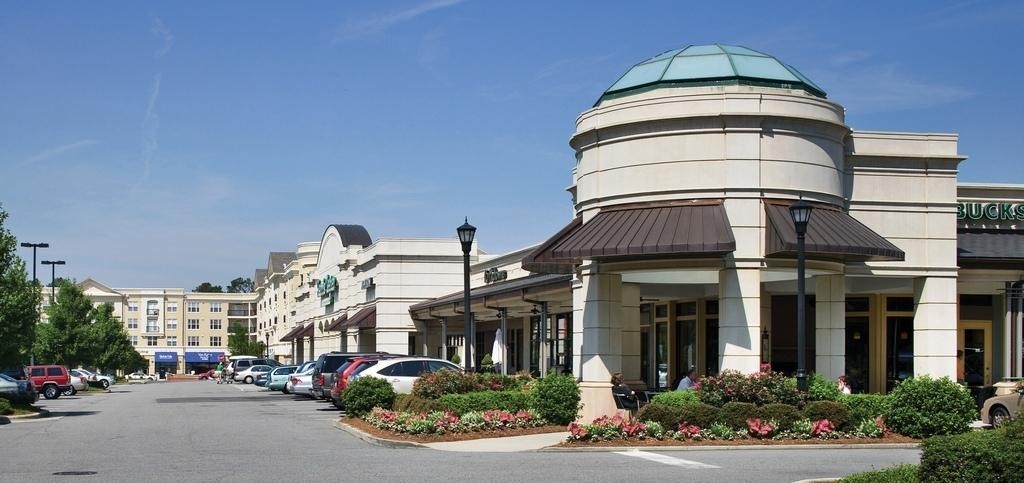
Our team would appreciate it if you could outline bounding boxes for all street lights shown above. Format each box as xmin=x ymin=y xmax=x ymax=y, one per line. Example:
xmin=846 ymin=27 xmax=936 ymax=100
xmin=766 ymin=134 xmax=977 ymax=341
xmin=21 ymin=241 xmax=50 ymax=282
xmin=40 ymin=259 xmax=66 ymax=300
xmin=786 ymin=189 xmax=814 ymax=388
xmin=265 ymin=333 xmax=270 ymax=358
xmin=457 ymin=215 xmax=478 ymax=373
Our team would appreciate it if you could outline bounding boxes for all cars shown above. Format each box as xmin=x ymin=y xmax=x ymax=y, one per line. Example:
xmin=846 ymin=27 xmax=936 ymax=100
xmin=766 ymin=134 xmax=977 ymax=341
xmin=124 ymin=372 xmax=157 ymax=382
xmin=980 ymin=388 xmax=1024 ymax=429
xmin=198 ymin=351 xmax=467 ymax=408
xmin=0 ymin=363 xmax=117 ymax=404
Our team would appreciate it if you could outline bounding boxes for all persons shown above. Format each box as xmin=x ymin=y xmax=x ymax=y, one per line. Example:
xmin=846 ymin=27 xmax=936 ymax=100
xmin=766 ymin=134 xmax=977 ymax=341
xmin=957 ymin=350 xmax=965 ymax=385
xmin=216 ymin=362 xmax=223 ymax=384
xmin=611 ymin=373 xmax=642 ymax=409
xmin=677 ymin=370 xmax=697 ymax=391
xmin=838 ymin=376 xmax=852 ymax=394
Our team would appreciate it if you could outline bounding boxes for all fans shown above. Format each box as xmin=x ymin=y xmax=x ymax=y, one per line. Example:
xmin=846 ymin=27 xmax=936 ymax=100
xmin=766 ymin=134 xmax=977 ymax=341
xmin=486 ymin=309 xmax=513 ymax=318
xmin=517 ymin=304 xmax=542 ymax=314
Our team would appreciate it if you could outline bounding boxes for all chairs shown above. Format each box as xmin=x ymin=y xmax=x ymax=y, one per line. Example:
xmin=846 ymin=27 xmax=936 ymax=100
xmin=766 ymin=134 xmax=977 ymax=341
xmin=612 ymin=386 xmax=667 ymax=417
